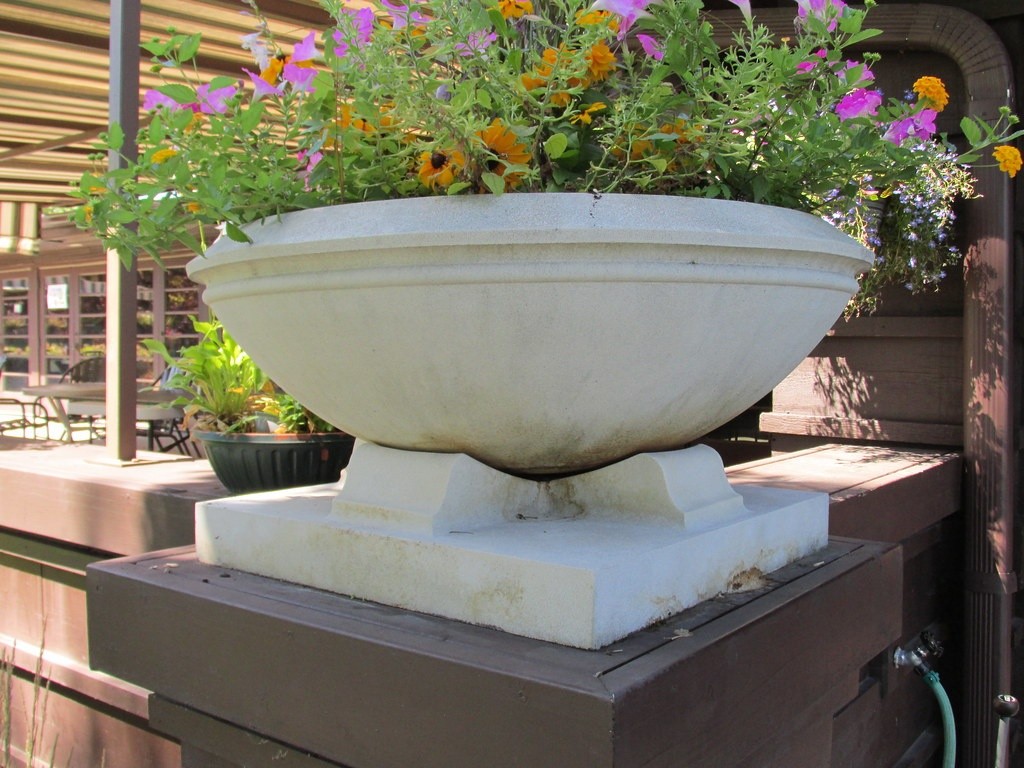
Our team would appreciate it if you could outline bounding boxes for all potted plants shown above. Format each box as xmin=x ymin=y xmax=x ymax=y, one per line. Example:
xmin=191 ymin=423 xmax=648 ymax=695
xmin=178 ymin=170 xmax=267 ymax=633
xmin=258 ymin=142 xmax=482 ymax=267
xmin=136 ymin=310 xmax=357 ymax=496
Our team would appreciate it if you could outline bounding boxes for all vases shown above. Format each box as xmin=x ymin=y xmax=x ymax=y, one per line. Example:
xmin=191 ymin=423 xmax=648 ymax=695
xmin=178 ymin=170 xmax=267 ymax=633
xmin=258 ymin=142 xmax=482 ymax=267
xmin=184 ymin=192 xmax=877 ymax=477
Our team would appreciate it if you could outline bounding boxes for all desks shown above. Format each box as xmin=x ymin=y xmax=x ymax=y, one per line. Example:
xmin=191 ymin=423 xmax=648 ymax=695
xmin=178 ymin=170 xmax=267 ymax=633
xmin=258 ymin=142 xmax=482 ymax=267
xmin=23 ymin=384 xmax=192 ymax=450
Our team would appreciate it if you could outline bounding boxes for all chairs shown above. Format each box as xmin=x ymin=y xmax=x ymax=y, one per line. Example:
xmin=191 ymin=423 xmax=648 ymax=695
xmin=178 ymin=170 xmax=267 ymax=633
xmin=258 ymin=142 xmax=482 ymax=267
xmin=34 ymin=357 xmax=106 ymax=444
xmin=135 ymin=366 xmax=203 ymax=458
xmin=0 ymin=398 xmax=49 ymax=440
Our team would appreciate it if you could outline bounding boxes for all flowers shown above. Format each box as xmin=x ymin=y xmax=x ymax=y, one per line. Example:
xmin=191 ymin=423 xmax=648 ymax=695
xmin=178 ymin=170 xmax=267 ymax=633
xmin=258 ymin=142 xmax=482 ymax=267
xmin=64 ymin=0 xmax=1024 ymax=321
